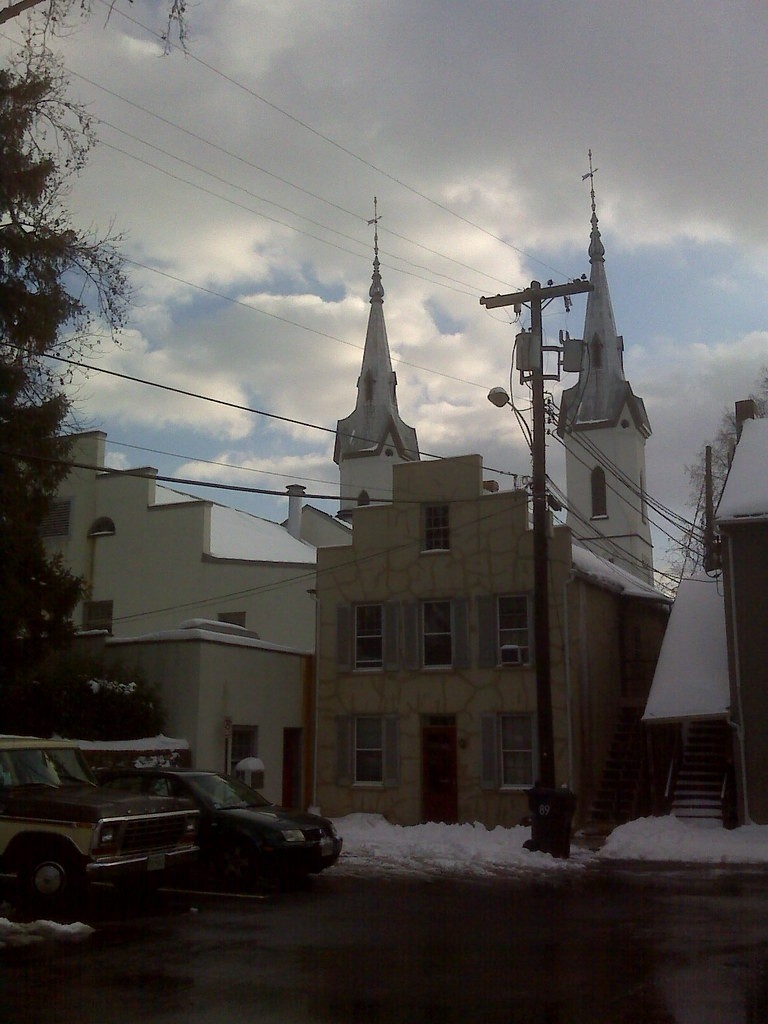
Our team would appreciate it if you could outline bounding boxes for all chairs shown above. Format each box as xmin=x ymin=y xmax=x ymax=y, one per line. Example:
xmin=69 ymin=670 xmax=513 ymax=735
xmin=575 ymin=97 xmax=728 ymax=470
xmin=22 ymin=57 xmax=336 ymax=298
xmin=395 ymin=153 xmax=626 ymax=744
xmin=141 ymin=778 xmax=195 ymax=798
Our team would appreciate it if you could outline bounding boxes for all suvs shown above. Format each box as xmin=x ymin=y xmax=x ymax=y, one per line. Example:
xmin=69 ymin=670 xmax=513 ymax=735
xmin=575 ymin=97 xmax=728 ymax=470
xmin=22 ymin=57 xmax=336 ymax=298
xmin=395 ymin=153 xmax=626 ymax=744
xmin=0 ymin=733 xmax=201 ymax=920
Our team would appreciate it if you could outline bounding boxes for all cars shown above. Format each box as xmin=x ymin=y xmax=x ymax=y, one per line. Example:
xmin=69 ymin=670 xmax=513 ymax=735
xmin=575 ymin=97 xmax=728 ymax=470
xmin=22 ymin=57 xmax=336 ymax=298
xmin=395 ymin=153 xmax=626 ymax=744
xmin=94 ymin=766 xmax=343 ymax=898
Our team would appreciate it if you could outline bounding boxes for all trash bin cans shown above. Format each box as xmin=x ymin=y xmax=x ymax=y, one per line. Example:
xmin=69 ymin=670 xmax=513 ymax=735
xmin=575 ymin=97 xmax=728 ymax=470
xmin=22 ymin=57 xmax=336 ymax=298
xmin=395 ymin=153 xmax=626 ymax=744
xmin=523 ymin=786 xmax=579 ymax=859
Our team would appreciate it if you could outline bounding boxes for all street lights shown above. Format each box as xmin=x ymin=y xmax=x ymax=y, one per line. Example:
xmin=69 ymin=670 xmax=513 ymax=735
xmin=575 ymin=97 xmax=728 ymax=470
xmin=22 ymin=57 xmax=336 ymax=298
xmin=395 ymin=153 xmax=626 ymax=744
xmin=477 ymin=276 xmax=600 ymax=862
